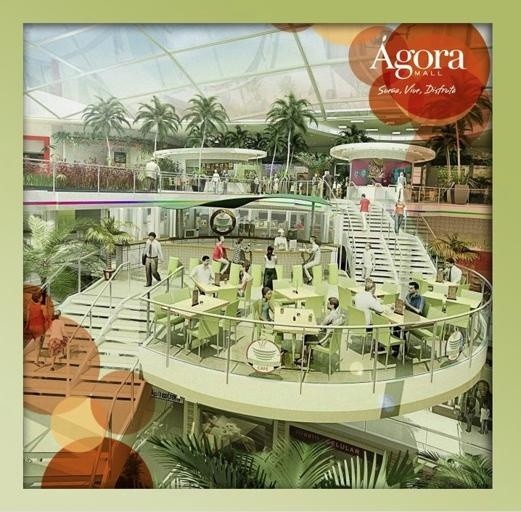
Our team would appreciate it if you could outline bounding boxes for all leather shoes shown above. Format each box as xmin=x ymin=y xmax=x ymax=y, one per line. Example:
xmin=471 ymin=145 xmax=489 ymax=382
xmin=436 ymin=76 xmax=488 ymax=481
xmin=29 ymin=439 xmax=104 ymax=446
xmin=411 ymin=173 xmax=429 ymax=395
xmin=143 ymin=283 xmax=152 ymax=287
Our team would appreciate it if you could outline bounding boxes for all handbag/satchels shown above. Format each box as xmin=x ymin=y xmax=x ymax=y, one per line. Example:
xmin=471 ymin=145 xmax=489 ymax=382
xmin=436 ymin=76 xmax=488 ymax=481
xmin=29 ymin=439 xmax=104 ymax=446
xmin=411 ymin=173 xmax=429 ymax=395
xmin=142 ymin=253 xmax=147 ymax=265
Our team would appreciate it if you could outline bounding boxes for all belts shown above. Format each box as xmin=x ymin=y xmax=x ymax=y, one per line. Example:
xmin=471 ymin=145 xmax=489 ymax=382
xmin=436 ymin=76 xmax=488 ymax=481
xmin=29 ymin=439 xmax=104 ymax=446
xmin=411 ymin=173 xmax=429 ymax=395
xmin=146 ymin=256 xmax=158 ymax=258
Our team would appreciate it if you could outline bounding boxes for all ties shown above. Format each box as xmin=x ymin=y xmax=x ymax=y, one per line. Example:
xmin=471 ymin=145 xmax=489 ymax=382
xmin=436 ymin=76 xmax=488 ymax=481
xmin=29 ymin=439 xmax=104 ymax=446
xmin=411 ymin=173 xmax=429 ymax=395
xmin=447 ymin=267 xmax=451 ymax=282
xmin=148 ymin=242 xmax=152 ymax=257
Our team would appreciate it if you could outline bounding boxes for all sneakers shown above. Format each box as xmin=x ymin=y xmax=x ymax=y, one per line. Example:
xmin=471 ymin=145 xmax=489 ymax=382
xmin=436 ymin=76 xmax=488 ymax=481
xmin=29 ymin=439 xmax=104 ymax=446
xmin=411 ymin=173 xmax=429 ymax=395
xmin=361 ymin=226 xmax=402 ymax=240
xmin=236 ymin=309 xmax=241 ymax=317
xmin=306 ymin=278 xmax=314 ymax=284
xmin=294 ymin=356 xmax=308 ymax=367
xmin=219 ymin=273 xmax=224 ymax=281
xmin=33 ymin=355 xmax=67 ymax=372
